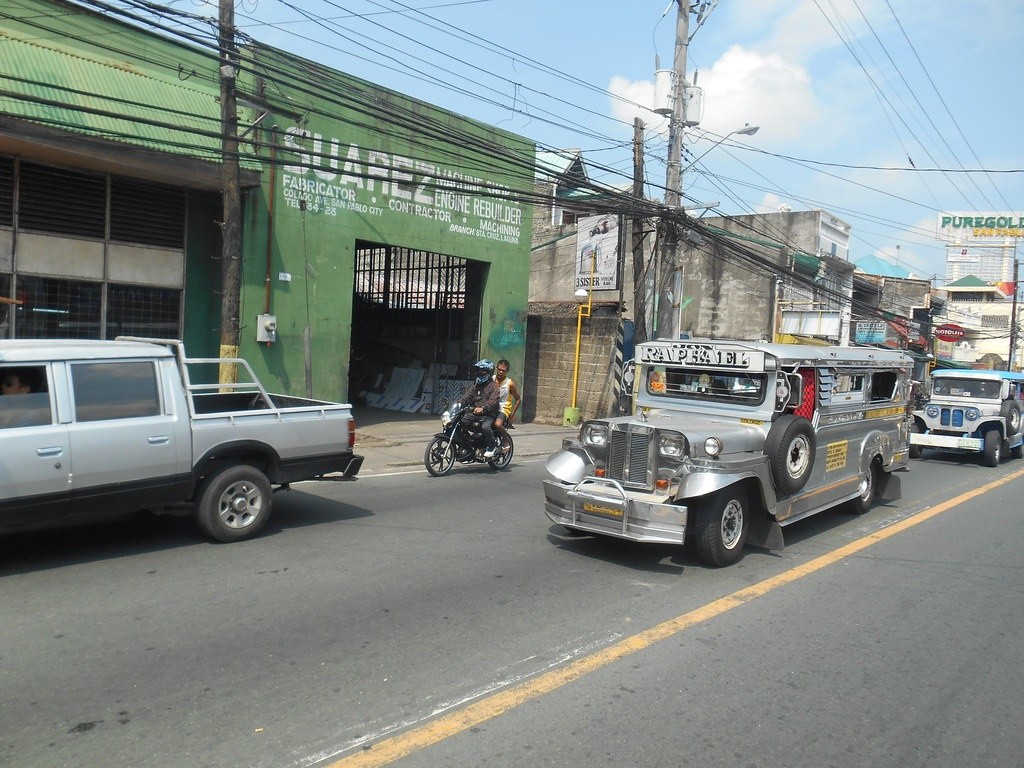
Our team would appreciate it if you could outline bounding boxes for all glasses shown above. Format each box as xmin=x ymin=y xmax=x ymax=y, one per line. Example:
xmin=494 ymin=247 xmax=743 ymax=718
xmin=2 ymin=380 xmax=17 ymax=388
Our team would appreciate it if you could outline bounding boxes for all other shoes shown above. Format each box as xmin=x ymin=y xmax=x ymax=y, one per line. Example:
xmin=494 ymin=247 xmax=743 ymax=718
xmin=483 ymin=447 xmax=497 ymax=457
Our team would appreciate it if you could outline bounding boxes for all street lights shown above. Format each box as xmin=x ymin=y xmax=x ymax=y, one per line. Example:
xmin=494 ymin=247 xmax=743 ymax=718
xmin=656 ymin=123 xmax=761 ymax=341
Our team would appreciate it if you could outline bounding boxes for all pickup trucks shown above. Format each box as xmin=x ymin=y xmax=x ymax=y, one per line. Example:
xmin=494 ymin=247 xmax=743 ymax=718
xmin=0 ymin=336 xmax=365 ymax=544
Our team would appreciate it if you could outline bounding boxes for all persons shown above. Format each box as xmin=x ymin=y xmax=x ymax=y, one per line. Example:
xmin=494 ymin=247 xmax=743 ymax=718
xmin=0 ymin=365 xmax=42 ymax=432
xmin=491 ymin=359 xmax=521 ymax=453
xmin=453 ymin=359 xmax=500 ymax=458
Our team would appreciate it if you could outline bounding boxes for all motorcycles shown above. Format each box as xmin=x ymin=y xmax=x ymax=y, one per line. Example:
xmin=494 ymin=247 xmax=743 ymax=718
xmin=424 ymin=402 xmax=516 ymax=477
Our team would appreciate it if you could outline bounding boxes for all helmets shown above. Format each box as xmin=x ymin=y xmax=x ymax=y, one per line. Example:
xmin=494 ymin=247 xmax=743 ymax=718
xmin=471 ymin=359 xmax=495 ymax=384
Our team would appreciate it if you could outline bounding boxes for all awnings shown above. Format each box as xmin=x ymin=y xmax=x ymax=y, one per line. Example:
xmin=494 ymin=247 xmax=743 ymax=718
xmin=929 ymin=357 xmax=972 ymax=370
xmin=877 ymin=343 xmax=929 ymax=363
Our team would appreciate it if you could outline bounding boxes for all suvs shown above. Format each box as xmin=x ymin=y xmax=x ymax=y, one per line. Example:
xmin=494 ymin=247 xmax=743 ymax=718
xmin=909 ymin=369 xmax=1024 ymax=467
xmin=543 ymin=338 xmax=915 ymax=568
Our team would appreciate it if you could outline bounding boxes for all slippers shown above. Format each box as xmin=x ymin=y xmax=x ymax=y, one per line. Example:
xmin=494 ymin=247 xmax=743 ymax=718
xmin=501 ymin=441 xmax=510 ymax=452
